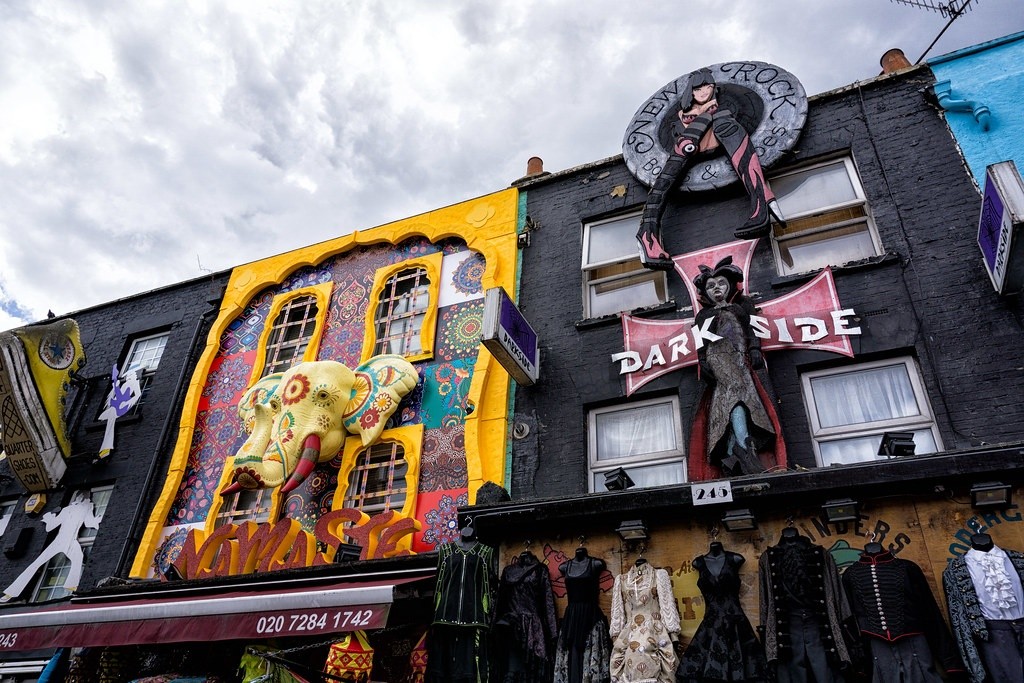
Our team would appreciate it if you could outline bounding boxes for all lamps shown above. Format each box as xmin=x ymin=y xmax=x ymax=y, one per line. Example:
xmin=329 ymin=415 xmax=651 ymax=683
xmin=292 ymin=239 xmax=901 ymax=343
xmin=616 ymin=519 xmax=648 ymax=539
xmin=603 ymin=466 xmax=636 ymax=492
xmin=821 ymin=498 xmax=862 ymax=525
xmin=722 ymin=510 xmax=759 ymax=532
xmin=968 ymin=481 xmax=1013 ymax=510
xmin=877 ymin=432 xmax=916 ymax=457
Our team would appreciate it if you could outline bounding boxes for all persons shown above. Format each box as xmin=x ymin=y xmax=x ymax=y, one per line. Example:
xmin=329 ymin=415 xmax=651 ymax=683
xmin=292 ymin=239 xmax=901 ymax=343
xmin=429 ymin=526 xmax=497 ymax=683
xmin=608 ymin=558 xmax=682 ymax=683
xmin=837 ymin=541 xmax=968 ymax=683
xmin=553 ymin=547 xmax=612 ymax=683
xmin=689 ymin=254 xmax=777 ymax=476
xmin=942 ymin=532 xmax=1024 ymax=683
xmin=673 ymin=541 xmax=767 ymax=682
xmin=489 ymin=550 xmax=559 ymax=683
xmin=756 ymin=527 xmax=853 ymax=683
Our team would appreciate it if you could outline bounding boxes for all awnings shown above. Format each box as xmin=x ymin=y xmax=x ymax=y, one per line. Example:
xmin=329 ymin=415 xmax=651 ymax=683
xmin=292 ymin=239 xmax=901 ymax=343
xmin=0 ymin=573 xmax=436 ymax=651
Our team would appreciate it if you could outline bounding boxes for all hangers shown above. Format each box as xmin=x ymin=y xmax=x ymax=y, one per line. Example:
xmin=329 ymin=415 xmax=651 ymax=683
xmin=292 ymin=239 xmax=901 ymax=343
xmin=455 ymin=516 xmax=799 ymax=565
